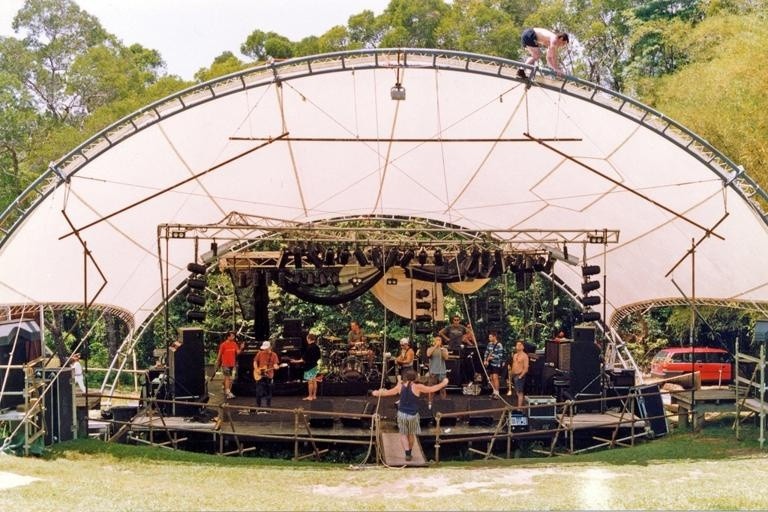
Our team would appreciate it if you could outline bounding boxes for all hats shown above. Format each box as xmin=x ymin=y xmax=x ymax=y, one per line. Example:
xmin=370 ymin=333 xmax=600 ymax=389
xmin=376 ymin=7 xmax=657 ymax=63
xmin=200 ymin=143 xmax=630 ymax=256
xmin=260 ymin=341 xmax=270 ymax=350
xmin=400 ymin=338 xmax=408 ymax=344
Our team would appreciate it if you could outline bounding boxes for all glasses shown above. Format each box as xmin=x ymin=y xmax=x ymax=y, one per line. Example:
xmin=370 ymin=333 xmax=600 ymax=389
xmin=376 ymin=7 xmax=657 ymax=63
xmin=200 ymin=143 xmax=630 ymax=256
xmin=454 ymin=319 xmax=459 ymax=321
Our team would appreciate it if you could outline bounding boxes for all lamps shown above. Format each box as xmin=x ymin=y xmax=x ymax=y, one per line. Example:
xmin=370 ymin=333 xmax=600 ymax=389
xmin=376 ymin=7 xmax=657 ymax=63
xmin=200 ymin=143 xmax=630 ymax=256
xmin=186 ymin=238 xmax=601 ymax=335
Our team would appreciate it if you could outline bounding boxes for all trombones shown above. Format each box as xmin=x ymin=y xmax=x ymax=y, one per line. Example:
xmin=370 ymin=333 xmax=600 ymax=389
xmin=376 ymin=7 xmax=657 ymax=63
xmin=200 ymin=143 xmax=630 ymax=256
xmin=505 ymin=363 xmax=513 ymax=396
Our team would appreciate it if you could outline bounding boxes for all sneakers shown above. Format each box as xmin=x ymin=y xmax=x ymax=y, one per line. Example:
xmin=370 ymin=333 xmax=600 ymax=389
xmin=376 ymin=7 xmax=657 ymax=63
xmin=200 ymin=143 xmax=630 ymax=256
xmin=488 ymin=393 xmax=501 ymax=400
xmin=406 ymin=456 xmax=412 ymax=461
xmin=225 ymin=391 xmax=236 ymax=399
xmin=516 ymin=69 xmax=528 ymax=78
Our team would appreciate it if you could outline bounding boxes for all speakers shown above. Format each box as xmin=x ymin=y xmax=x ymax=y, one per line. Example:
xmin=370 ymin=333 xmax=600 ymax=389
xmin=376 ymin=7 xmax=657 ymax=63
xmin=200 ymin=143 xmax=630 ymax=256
xmin=310 ymin=398 xmax=493 ymax=428
xmin=167 ymin=328 xmax=205 ymax=416
xmin=570 ymin=326 xmax=602 ymax=414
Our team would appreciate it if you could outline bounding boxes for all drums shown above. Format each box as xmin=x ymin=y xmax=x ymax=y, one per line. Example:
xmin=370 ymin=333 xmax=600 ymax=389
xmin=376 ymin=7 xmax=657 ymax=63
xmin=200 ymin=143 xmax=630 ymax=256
xmin=354 ymin=341 xmax=371 ymax=357
xmin=340 ymin=356 xmax=364 ymax=384
xmin=328 ymin=343 xmax=350 ymax=367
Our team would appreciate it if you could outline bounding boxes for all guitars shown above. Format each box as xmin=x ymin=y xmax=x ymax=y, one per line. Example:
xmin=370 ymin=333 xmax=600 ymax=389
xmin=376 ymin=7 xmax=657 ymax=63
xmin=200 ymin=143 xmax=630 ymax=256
xmin=485 ymin=349 xmax=494 ymax=369
xmin=252 ymin=362 xmax=289 ymax=380
xmin=306 ymin=331 xmax=326 ymax=345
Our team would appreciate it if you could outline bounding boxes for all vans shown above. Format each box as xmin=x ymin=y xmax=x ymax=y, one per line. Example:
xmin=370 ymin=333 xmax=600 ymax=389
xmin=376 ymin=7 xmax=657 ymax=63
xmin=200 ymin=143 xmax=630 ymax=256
xmin=650 ymin=347 xmax=732 ymax=386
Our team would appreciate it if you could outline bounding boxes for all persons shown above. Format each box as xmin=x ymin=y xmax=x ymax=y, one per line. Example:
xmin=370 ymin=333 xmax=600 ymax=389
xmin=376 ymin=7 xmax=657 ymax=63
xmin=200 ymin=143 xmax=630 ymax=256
xmin=214 ymin=331 xmax=245 ymax=399
xmin=427 ymin=316 xmax=529 ymax=412
xmin=517 ymin=28 xmax=569 ymax=79
xmin=348 ymin=320 xmax=365 ymax=346
xmin=372 ymin=370 xmax=447 ymax=460
xmin=70 ymin=354 xmax=86 ymax=393
xmin=396 ymin=338 xmax=414 ymax=371
xmin=289 ymin=334 xmax=321 ymax=401
xmin=253 ymin=341 xmax=279 ymax=407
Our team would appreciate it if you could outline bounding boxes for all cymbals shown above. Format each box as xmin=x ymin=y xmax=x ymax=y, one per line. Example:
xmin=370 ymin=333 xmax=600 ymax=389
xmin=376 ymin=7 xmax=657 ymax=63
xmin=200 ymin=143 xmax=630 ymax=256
xmin=367 ymin=340 xmax=383 ymax=345
xmin=323 ymin=335 xmax=341 ymax=340
